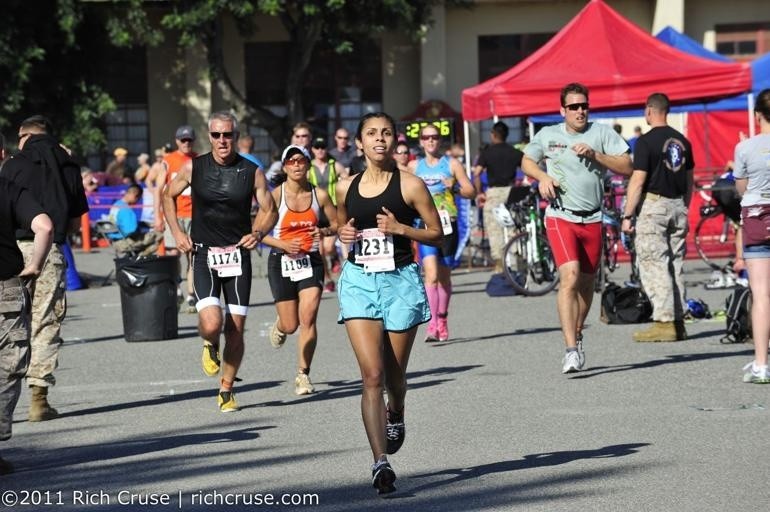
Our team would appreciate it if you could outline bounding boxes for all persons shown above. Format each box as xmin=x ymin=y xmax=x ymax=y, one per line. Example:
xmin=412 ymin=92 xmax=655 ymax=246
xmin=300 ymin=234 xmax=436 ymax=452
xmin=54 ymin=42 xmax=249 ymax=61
xmin=406 ymin=122 xmax=476 ymax=343
xmin=1 ymin=133 xmax=56 ymax=477
xmin=77 ymin=120 xmax=745 ymax=320
xmin=522 ymin=82 xmax=636 ymax=377
xmin=332 ymin=111 xmax=447 ymax=495
xmin=1 ymin=116 xmax=82 ymax=421
xmin=162 ymin=111 xmax=279 ymax=414
xmin=618 ymin=92 xmax=697 ymax=345
xmin=251 ymin=142 xmax=337 ymax=395
xmin=731 ymin=87 xmax=770 ymax=385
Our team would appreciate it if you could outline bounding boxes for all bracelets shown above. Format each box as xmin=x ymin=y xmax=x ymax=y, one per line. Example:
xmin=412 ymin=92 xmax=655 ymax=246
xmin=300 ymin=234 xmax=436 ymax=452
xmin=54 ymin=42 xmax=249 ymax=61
xmin=254 ymin=229 xmax=264 ymax=240
xmin=621 ymin=215 xmax=635 ymax=220
xmin=323 ymin=226 xmax=334 ymax=236
xmin=451 ymin=182 xmax=461 ymax=193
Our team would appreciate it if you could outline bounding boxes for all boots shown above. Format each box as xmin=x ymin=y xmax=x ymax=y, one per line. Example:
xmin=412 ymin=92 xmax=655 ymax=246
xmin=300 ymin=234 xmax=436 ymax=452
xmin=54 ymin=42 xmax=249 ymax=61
xmin=28 ymin=385 xmax=59 ymax=423
xmin=0 ymin=455 xmax=13 ymax=478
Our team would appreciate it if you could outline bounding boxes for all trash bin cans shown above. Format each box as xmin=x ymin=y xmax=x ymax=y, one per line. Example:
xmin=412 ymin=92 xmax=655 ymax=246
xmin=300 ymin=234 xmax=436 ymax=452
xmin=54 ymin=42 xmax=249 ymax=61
xmin=113 ymin=255 xmax=179 ymax=342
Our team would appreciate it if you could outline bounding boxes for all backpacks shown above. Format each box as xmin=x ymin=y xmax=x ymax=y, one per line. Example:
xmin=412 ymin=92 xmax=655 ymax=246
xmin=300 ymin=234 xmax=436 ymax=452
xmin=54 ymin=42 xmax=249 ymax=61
xmin=719 ymin=285 xmax=751 ymax=345
xmin=599 ymin=283 xmax=653 ymax=326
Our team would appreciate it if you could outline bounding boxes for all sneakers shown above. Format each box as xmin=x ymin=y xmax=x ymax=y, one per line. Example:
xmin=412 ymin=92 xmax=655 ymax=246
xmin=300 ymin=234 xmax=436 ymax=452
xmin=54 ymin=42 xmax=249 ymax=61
xmin=577 ymin=340 xmax=585 ymax=368
xmin=384 ymin=404 xmax=407 ymax=455
xmin=704 ymin=269 xmax=725 ymax=290
xmin=634 ymin=321 xmax=677 ymax=342
xmin=723 ymin=267 xmax=739 ymax=290
xmin=174 ymin=274 xmax=337 ymax=413
xmin=424 ymin=325 xmax=439 ymax=343
xmin=437 ymin=315 xmax=450 ymax=342
xmin=743 ymin=361 xmax=770 ymax=385
xmin=371 ymin=458 xmax=398 ymax=498
xmin=561 ymin=351 xmax=582 ymax=374
xmin=676 ymin=323 xmax=689 ymax=342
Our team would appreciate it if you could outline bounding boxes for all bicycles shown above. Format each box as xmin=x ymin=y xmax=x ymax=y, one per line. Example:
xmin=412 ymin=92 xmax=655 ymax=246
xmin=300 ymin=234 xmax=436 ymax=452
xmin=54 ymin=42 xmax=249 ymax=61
xmin=501 ymin=174 xmax=625 ymax=298
xmin=694 ymin=172 xmax=740 ymax=271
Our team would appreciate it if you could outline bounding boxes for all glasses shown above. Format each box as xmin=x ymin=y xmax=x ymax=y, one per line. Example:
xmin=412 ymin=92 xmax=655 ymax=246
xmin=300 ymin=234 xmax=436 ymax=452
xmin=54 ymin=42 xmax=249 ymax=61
xmin=16 ymin=133 xmax=31 ymax=144
xmin=337 ymin=136 xmax=349 ymax=140
xmin=395 ymin=151 xmax=410 ymax=155
xmin=284 ymin=158 xmax=309 ymax=165
xmin=420 ymin=135 xmax=440 ymax=140
xmin=210 ymin=131 xmax=236 ymax=139
xmin=181 ymin=137 xmax=194 ymax=143
xmin=295 ymin=134 xmax=308 ymax=138
xmin=564 ymin=103 xmax=589 ymax=110
xmin=313 ymin=145 xmax=327 ymax=150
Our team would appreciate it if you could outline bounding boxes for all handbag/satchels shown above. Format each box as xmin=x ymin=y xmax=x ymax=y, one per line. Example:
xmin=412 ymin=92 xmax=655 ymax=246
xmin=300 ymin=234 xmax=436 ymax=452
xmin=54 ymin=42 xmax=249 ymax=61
xmin=487 ymin=267 xmax=526 ymax=297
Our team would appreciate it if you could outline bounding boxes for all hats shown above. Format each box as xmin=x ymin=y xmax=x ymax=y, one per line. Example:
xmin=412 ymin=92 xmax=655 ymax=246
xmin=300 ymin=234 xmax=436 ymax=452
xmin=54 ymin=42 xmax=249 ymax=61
xmin=312 ymin=137 xmax=327 ymax=147
xmin=282 ymin=144 xmax=312 ymax=164
xmin=114 ymin=147 xmax=128 ymax=158
xmin=176 ymin=125 xmax=195 ymax=141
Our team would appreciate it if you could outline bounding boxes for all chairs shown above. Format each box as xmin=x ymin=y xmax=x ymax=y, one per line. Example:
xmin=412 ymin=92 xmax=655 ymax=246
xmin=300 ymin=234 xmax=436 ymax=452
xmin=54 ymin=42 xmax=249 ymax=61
xmin=98 ymin=221 xmax=158 ymax=284
